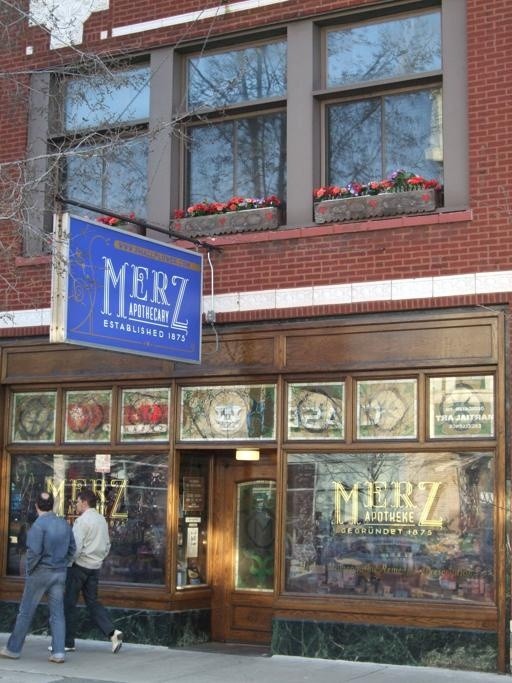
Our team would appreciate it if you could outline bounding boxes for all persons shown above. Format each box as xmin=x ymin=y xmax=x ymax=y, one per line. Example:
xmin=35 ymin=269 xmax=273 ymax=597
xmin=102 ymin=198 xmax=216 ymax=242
xmin=0 ymin=493 xmax=76 ymax=663
xmin=47 ymin=489 xmax=124 ymax=653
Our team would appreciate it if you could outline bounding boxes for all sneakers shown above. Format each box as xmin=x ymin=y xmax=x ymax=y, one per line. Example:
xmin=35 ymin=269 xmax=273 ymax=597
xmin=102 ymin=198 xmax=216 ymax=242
xmin=47 ymin=654 xmax=64 ymax=663
xmin=109 ymin=629 xmax=124 ymax=653
xmin=48 ymin=644 xmax=76 ymax=652
xmin=0 ymin=646 xmax=21 ymax=659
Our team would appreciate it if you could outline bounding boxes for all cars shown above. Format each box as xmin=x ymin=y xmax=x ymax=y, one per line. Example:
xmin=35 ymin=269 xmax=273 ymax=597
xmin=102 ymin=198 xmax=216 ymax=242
xmin=321 ymin=534 xmax=430 ymax=592
xmin=102 ymin=517 xmax=163 ymax=578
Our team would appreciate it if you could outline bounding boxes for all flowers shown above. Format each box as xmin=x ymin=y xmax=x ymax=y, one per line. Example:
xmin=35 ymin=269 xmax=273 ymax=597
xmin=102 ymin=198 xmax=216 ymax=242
xmin=173 ymin=195 xmax=281 ymax=220
xmin=93 ymin=211 xmax=136 ymax=226
xmin=313 ymin=169 xmax=444 ymax=201
